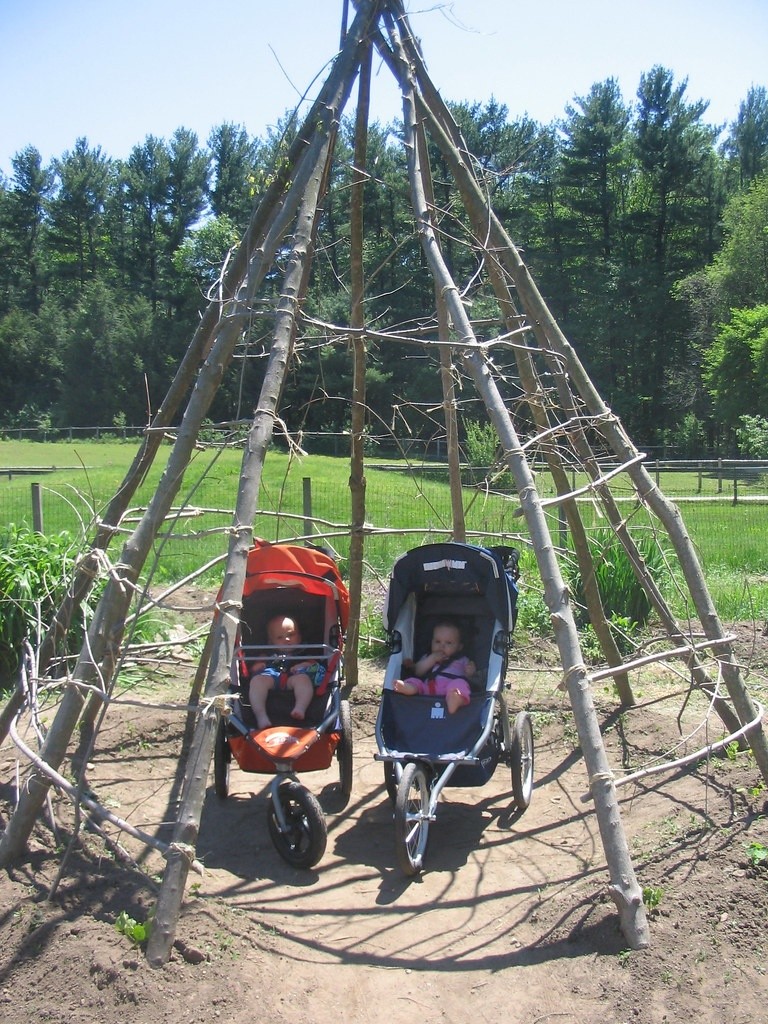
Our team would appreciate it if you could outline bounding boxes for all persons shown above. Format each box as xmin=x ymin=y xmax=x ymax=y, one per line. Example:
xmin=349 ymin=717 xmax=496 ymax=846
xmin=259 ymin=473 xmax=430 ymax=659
xmin=250 ymin=614 xmax=316 ymax=729
xmin=392 ymin=616 xmax=476 ymax=714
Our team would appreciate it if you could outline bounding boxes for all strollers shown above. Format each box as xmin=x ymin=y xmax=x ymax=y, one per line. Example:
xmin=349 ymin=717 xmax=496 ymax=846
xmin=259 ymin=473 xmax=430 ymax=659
xmin=196 ymin=542 xmax=356 ymax=871
xmin=375 ymin=540 xmax=538 ymax=875
